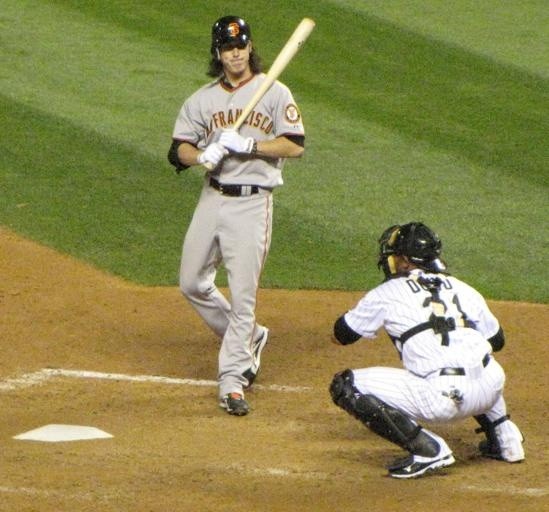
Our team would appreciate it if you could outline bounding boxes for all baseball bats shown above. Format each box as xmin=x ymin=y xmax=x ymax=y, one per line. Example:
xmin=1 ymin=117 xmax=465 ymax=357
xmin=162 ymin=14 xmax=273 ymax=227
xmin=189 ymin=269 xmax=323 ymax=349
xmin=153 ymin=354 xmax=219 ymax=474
xmin=202 ymin=17 xmax=317 ymax=172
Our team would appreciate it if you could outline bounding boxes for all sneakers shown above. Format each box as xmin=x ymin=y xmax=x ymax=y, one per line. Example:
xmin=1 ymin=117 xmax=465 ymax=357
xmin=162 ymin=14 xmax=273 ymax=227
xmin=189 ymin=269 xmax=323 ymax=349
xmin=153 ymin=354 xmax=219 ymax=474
xmin=388 ymin=439 xmax=457 ymax=479
xmin=218 ymin=391 xmax=248 ymax=416
xmin=479 ymin=439 xmax=525 ymax=462
xmin=244 ymin=324 xmax=269 ymax=385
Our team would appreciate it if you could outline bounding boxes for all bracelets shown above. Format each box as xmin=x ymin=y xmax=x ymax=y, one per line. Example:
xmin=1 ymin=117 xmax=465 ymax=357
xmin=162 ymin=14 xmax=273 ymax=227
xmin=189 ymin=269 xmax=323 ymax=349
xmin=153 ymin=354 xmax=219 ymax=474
xmin=251 ymin=138 xmax=258 ymax=161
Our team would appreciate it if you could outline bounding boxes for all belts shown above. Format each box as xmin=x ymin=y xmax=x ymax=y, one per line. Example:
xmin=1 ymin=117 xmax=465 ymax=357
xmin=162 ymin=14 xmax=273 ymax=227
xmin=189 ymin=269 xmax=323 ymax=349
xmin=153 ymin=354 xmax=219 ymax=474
xmin=210 ymin=178 xmax=259 ymax=196
xmin=442 ymin=355 xmax=493 ymax=376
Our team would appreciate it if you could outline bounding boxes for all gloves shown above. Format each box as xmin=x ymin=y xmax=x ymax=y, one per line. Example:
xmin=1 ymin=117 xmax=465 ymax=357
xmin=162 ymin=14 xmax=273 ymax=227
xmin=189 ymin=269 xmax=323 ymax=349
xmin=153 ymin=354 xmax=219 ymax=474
xmin=219 ymin=126 xmax=255 ymax=154
xmin=198 ymin=143 xmax=228 ymax=165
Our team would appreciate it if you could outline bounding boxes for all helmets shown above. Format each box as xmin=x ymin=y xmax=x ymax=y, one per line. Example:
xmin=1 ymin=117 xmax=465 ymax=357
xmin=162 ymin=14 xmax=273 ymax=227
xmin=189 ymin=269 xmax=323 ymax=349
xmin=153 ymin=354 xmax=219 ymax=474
xmin=380 ymin=221 xmax=450 ymax=276
xmin=211 ymin=15 xmax=251 ymax=48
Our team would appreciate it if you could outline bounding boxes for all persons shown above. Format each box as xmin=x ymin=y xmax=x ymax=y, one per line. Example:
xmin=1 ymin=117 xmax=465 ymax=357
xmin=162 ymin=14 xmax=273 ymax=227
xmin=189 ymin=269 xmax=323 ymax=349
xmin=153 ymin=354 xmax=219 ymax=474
xmin=326 ymin=221 xmax=527 ymax=478
xmin=167 ymin=15 xmax=305 ymax=415
